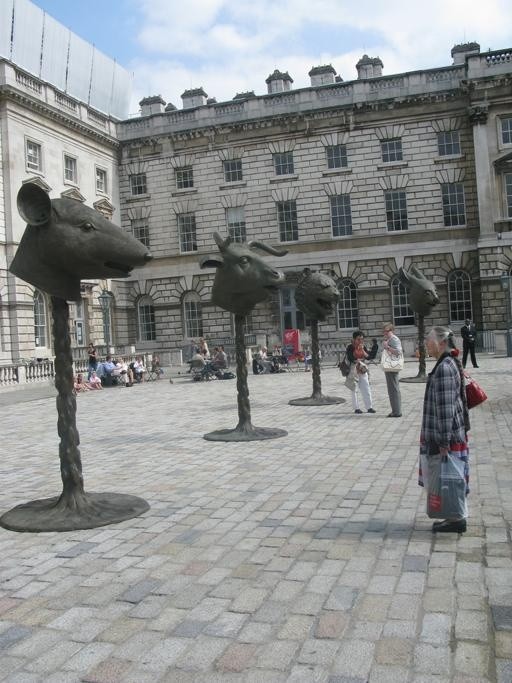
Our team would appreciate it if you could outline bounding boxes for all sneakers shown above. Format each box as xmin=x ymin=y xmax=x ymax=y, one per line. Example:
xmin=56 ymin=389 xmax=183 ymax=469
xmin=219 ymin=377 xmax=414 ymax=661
xmin=355 ymin=409 xmax=362 ymax=413
xmin=368 ymin=408 xmax=375 ymax=413
xmin=433 ymin=519 xmax=466 ymax=532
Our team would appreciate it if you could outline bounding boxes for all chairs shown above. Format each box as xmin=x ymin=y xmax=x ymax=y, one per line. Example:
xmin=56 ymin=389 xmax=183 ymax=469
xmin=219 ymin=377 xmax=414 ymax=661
xmin=188 ymin=358 xmax=228 ymax=380
xmin=100 ymin=360 xmax=159 ymax=382
xmin=253 ymin=353 xmax=301 ymax=375
xmin=366 ymin=349 xmax=378 ymax=366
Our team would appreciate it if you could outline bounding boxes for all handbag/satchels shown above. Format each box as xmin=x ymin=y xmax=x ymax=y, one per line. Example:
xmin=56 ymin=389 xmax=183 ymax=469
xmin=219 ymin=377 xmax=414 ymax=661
xmin=466 ymin=381 xmax=487 ymax=409
xmin=381 ymin=349 xmax=404 ymax=372
xmin=340 ymin=362 xmax=349 ymax=377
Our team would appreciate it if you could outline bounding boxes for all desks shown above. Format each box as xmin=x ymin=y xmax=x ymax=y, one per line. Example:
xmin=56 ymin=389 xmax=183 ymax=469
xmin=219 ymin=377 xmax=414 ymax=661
xmin=331 ymin=350 xmax=347 ymax=368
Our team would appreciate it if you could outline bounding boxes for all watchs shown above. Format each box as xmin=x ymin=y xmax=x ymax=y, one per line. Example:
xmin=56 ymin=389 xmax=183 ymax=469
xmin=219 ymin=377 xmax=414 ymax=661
xmin=387 ymin=345 xmax=390 ymax=350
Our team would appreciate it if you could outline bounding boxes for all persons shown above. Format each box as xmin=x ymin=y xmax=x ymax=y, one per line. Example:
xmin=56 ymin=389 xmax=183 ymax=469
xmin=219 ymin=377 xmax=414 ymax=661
xmin=420 ymin=323 xmax=472 ymax=534
xmin=74 ymin=336 xmax=323 ymax=393
xmin=460 ymin=318 xmax=479 ymax=369
xmin=378 ymin=321 xmax=404 ymax=418
xmin=344 ymin=330 xmax=378 ymax=414
